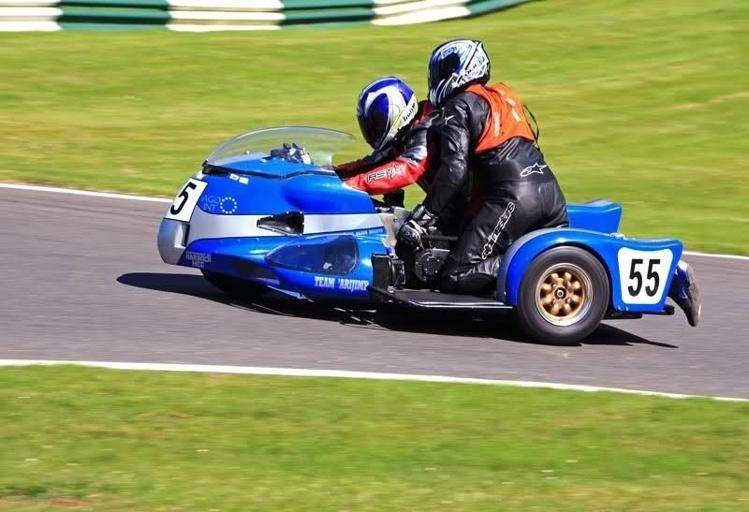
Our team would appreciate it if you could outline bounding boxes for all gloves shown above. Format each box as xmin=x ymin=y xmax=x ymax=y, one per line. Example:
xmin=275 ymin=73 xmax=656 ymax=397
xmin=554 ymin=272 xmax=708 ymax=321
xmin=399 ymin=205 xmax=440 ymax=246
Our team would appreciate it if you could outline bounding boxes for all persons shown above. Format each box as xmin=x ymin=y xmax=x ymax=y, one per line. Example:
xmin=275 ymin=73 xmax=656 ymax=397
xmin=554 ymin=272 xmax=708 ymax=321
xmin=320 ymin=75 xmax=484 ymax=236
xmin=398 ymin=38 xmax=701 ymax=327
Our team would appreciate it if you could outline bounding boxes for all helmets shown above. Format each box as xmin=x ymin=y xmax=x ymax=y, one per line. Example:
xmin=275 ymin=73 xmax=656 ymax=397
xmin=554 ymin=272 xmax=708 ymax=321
xmin=427 ymin=38 xmax=492 ymax=107
xmin=357 ymin=74 xmax=420 ymax=152
